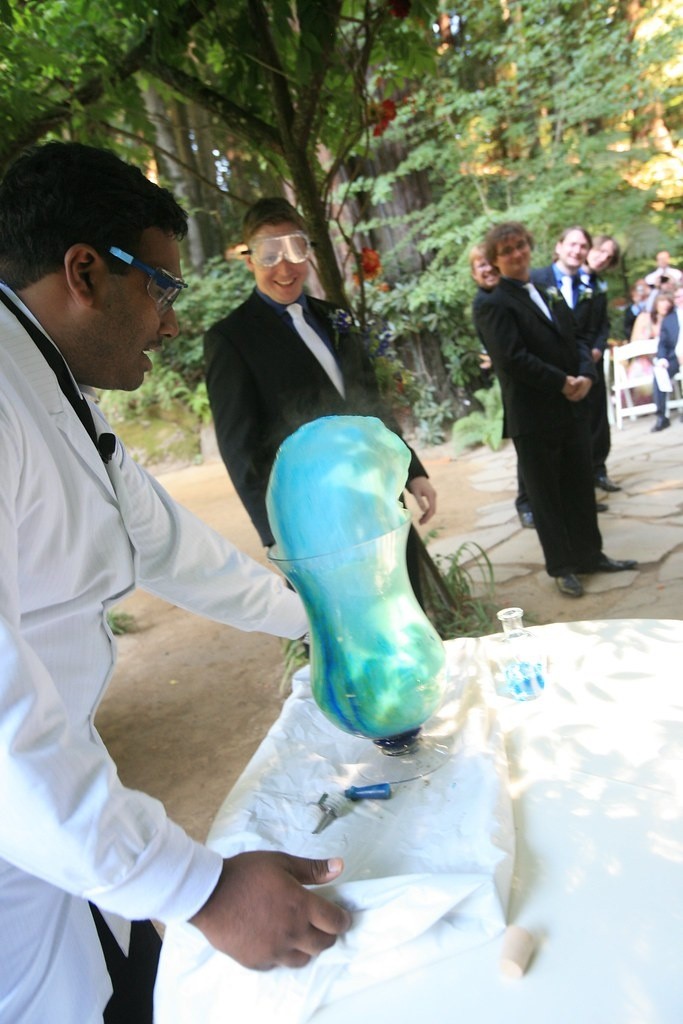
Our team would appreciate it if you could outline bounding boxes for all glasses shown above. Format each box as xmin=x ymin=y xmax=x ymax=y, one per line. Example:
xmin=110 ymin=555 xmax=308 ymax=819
xmin=57 ymin=244 xmax=189 ymax=314
xmin=493 ymin=239 xmax=530 ymax=259
xmin=250 ymin=231 xmax=316 ymax=269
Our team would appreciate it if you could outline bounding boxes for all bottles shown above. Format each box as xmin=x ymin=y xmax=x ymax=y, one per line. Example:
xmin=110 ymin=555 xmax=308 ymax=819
xmin=496 ymin=607 xmax=548 ymax=702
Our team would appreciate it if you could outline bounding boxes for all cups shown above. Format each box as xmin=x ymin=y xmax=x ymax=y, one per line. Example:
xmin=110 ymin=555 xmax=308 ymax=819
xmin=266 ymin=510 xmax=458 ymax=784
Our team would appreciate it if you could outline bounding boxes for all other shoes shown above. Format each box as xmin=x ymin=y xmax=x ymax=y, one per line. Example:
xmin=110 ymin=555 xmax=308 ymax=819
xmin=652 ymin=419 xmax=670 ymax=432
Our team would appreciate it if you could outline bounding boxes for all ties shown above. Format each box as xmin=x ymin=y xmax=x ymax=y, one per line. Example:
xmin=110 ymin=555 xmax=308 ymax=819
xmin=560 ymin=275 xmax=575 ymax=308
xmin=287 ymin=301 xmax=345 ymax=402
xmin=523 ymin=282 xmax=552 ymax=320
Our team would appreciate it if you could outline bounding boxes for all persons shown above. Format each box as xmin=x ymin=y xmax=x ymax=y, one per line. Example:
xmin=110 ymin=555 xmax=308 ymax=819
xmin=0 ymin=140 xmax=353 ymax=1024
xmin=625 ymin=251 xmax=683 ymax=431
xmin=469 ymin=219 xmax=638 ymax=596
xmin=204 ymin=197 xmax=435 ymax=614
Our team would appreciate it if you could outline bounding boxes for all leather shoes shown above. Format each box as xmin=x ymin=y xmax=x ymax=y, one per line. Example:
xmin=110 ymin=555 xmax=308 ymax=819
xmin=594 ymin=475 xmax=620 ymax=493
xmin=578 ymin=553 xmax=638 ymax=573
xmin=555 ymin=572 xmax=585 ymax=599
xmin=594 ymin=503 xmax=609 ymax=513
xmin=520 ymin=510 xmax=538 ymax=530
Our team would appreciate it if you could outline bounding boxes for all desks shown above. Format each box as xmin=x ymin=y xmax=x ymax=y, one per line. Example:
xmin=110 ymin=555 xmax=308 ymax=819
xmin=150 ymin=617 xmax=683 ymax=1024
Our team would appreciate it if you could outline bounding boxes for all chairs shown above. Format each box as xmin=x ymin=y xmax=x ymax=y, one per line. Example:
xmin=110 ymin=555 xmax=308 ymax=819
xmin=602 ymin=337 xmax=683 ymax=430
